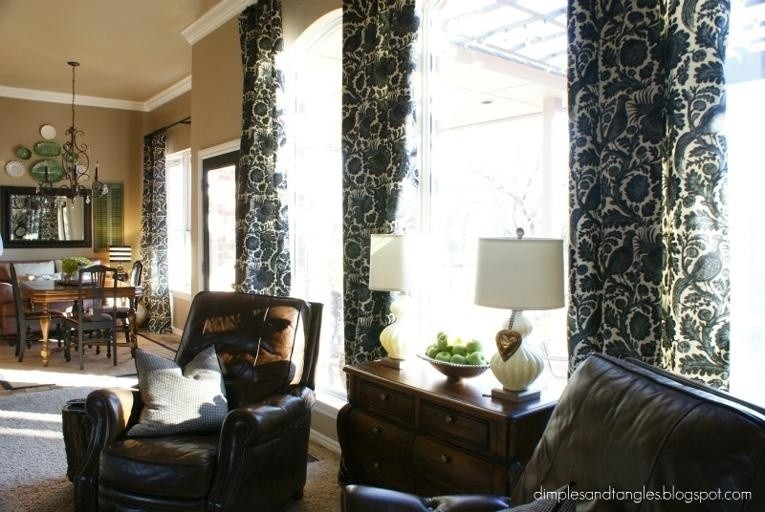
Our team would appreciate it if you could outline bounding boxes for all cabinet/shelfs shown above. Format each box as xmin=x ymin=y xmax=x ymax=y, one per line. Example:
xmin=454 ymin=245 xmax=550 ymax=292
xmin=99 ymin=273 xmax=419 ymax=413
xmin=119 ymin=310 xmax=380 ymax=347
xmin=337 ymin=361 xmax=561 ymax=497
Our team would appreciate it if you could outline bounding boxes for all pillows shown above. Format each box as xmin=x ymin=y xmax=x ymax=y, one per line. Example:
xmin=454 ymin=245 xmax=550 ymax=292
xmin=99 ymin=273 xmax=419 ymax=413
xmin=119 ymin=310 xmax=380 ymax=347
xmin=54 ymin=260 xmax=101 ymax=280
xmin=126 ymin=344 xmax=229 ymax=437
xmin=6 ymin=261 xmax=55 ymax=283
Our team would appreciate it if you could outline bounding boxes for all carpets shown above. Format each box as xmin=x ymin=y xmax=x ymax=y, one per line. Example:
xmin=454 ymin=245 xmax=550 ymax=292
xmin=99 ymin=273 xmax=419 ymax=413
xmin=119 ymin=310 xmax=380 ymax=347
xmin=0 ymin=386 xmax=341 ymax=512
xmin=0 ymin=330 xmax=178 ymax=391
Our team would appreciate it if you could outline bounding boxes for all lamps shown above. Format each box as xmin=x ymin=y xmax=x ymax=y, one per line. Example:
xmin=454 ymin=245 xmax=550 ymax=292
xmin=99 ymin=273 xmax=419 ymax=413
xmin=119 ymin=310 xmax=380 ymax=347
xmin=36 ymin=62 xmax=109 ymax=207
xmin=108 ymin=245 xmax=132 ymax=282
xmin=475 ymin=229 xmax=564 ymax=404
xmin=369 ymin=233 xmax=413 ymax=371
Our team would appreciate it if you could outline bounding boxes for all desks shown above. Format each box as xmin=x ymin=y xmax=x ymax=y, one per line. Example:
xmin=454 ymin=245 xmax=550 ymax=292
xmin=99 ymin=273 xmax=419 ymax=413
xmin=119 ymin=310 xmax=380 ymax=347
xmin=21 ymin=277 xmax=138 ymax=366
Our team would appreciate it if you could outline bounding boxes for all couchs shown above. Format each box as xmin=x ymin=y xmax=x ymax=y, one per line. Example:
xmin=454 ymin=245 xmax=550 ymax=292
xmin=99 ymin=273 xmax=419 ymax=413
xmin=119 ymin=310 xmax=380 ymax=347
xmin=341 ymin=352 xmax=765 ymax=512
xmin=0 ymin=258 xmax=129 ymax=346
xmin=72 ymin=290 xmax=322 ymax=512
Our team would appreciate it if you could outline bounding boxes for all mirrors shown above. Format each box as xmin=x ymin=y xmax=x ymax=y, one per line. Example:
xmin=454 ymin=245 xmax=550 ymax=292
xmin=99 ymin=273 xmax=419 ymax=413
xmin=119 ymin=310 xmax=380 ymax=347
xmin=0 ymin=185 xmax=92 ymax=248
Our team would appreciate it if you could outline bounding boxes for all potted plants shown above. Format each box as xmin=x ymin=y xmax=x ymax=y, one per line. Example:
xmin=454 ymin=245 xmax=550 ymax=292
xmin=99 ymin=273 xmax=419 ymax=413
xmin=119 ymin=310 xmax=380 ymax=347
xmin=62 ymin=257 xmax=91 ymax=281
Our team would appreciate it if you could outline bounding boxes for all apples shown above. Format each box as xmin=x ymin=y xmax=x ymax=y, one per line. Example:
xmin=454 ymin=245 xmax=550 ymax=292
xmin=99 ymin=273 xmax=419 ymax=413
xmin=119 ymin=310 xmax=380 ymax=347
xmin=427 ymin=331 xmax=487 ymax=368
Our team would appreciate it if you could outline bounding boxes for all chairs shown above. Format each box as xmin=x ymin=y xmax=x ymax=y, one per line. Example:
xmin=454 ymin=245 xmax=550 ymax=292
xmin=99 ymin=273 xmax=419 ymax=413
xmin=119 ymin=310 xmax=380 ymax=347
xmin=90 ymin=261 xmax=143 ymax=357
xmin=64 ymin=265 xmax=118 ymax=370
xmin=9 ymin=264 xmax=70 ymax=363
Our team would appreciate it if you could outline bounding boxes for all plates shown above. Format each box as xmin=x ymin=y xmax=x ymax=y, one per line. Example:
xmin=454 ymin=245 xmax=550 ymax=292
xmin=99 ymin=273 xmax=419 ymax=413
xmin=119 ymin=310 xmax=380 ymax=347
xmin=30 ymin=159 xmax=65 ymax=183
xmin=65 ymin=151 xmax=79 ymax=162
xmin=5 ymin=160 xmax=25 ymax=179
xmin=70 ymin=164 xmax=88 ymax=182
xmin=40 ymin=124 xmax=56 ymax=139
xmin=16 ymin=146 xmax=32 ymax=161
xmin=14 ymin=225 xmax=26 ymax=239
xmin=33 ymin=141 xmax=61 ymax=157
